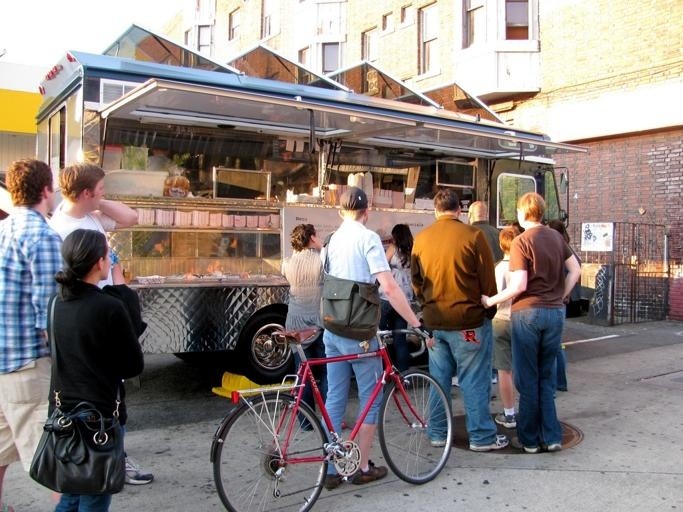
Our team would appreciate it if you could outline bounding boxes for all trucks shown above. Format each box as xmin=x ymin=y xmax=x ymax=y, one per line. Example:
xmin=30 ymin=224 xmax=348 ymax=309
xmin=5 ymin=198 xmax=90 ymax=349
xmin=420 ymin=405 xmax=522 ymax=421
xmin=30 ymin=49 xmax=592 ymax=385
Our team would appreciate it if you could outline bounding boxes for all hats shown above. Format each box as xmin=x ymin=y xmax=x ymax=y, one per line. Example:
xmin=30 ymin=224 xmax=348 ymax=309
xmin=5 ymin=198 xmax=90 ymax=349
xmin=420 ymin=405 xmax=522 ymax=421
xmin=340 ymin=186 xmax=368 ymax=210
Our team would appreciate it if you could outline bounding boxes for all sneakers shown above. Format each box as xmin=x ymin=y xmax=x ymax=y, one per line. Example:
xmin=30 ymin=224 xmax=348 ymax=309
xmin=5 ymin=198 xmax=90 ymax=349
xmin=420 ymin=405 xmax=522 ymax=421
xmin=124 ymin=457 xmax=153 ymax=485
xmin=324 ymin=460 xmax=388 ymax=489
xmin=431 ymin=414 xmax=562 ymax=453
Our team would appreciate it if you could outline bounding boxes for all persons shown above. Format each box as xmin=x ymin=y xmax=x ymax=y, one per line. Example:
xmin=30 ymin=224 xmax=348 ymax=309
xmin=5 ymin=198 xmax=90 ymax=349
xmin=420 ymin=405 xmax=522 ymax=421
xmin=316 ymin=187 xmax=435 ymax=491
xmin=479 ymin=192 xmax=581 ymax=453
xmin=410 ymin=190 xmax=511 ymax=453
xmin=546 ymin=219 xmax=570 ymax=396
xmin=378 ymin=223 xmax=414 ymax=375
xmin=468 ymin=202 xmax=504 ymax=383
xmin=46 ymin=228 xmax=141 ymax=511
xmin=45 ymin=165 xmax=153 ymax=485
xmin=283 ymin=223 xmax=347 ymax=432
xmin=0 ymin=159 xmax=69 ymax=511
xmin=493 ymin=227 xmax=519 ymax=427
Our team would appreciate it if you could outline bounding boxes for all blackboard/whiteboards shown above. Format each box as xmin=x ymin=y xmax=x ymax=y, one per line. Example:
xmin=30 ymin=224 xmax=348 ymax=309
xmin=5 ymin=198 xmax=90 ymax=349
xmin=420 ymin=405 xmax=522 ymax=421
xmin=212 ymin=166 xmax=272 ymax=203
xmin=436 ymin=160 xmax=476 ymax=190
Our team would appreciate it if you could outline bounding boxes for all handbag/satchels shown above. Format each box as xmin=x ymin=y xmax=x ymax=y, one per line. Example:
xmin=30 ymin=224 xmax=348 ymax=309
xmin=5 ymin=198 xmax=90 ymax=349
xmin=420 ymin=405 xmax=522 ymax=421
xmin=29 ymin=401 xmax=125 ymax=495
xmin=319 ymin=273 xmax=382 ymax=340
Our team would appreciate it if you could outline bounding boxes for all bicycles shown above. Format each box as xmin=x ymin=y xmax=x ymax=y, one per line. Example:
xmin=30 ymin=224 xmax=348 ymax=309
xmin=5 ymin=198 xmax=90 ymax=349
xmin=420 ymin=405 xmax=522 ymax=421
xmin=208 ymin=325 xmax=455 ymax=510
